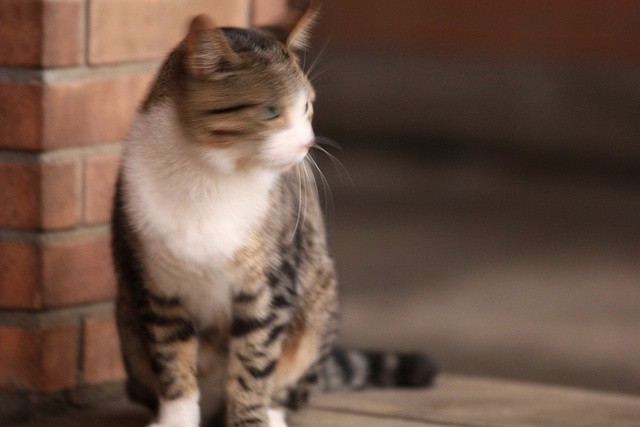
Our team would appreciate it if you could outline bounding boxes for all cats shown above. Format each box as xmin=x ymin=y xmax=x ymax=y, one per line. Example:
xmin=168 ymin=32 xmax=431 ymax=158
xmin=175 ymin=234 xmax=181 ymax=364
xmin=111 ymin=1 xmax=437 ymax=427
